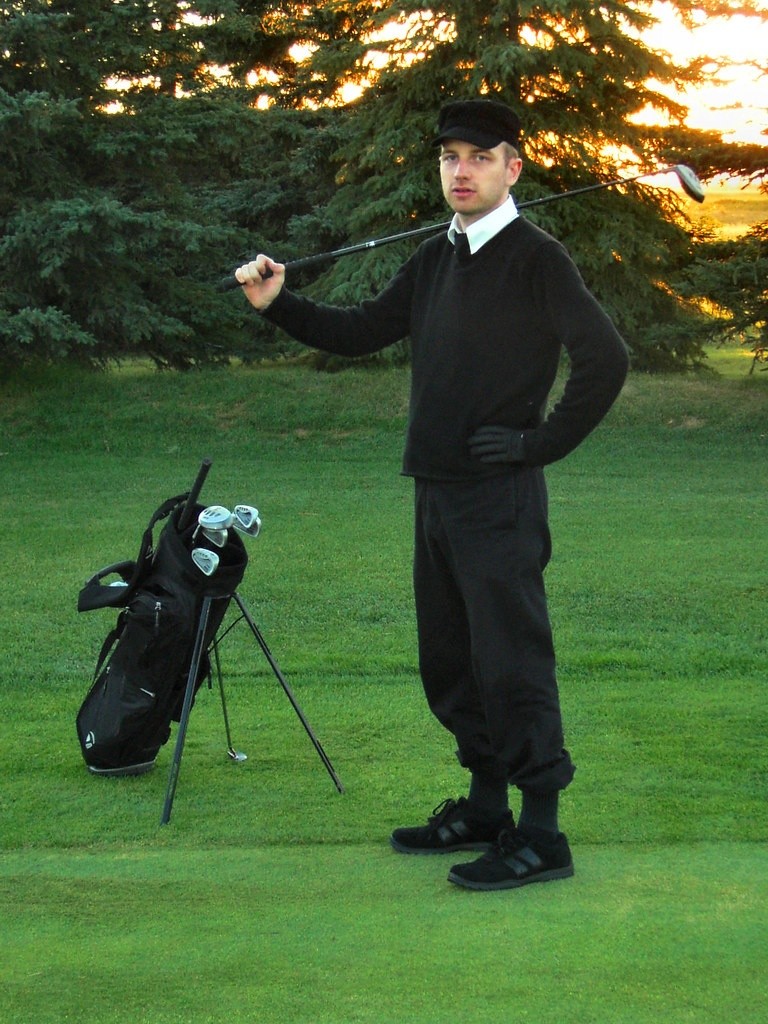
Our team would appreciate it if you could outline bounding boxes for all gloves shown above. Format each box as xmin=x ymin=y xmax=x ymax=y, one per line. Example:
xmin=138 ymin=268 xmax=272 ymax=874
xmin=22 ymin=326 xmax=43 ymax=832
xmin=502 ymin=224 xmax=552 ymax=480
xmin=466 ymin=425 xmax=545 ymax=465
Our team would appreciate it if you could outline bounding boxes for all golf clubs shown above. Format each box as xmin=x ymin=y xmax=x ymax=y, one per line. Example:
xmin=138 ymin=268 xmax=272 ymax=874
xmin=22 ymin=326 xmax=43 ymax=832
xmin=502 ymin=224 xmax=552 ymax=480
xmin=217 ymin=161 xmax=706 ymax=294
xmin=176 ymin=458 xmax=262 ymax=577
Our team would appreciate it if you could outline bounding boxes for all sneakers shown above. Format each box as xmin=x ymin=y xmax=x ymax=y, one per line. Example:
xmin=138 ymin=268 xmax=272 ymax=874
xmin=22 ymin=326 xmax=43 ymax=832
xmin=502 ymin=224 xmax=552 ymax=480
xmin=389 ymin=796 xmax=517 ymax=855
xmin=447 ymin=826 xmax=575 ymax=891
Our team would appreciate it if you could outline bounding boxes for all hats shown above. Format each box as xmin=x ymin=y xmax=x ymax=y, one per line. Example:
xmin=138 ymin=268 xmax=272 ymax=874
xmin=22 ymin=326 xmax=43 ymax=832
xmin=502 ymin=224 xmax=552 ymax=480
xmin=429 ymin=99 xmax=522 ymax=156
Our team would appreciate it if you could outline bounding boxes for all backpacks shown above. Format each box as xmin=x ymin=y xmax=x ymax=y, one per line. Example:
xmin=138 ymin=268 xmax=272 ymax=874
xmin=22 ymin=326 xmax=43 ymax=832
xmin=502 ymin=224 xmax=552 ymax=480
xmin=74 ymin=491 xmax=248 ymax=776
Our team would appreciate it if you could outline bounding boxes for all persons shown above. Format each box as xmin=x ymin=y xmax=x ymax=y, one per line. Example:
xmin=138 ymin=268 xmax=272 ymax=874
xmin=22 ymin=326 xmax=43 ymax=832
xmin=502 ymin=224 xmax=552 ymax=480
xmin=230 ymin=97 xmax=630 ymax=893
